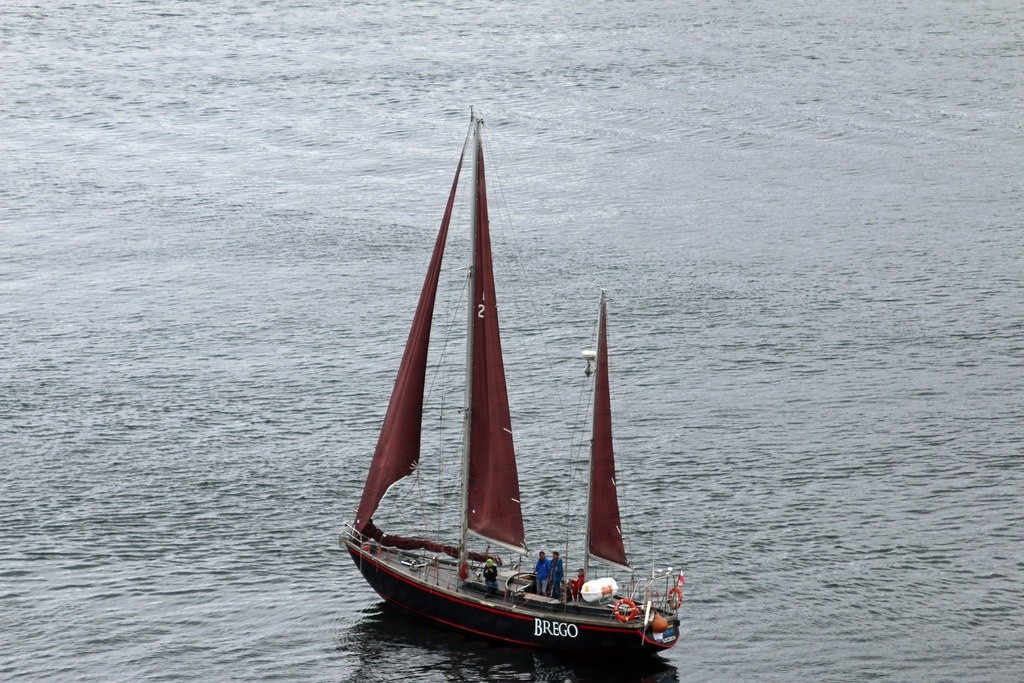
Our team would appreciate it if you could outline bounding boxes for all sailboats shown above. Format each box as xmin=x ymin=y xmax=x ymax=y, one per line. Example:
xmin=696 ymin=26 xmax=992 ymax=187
xmin=339 ymin=104 xmax=684 ymax=658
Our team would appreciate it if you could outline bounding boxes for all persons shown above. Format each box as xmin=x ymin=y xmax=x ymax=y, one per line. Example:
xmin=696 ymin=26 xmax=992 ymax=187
xmin=536 ymin=551 xmax=551 ymax=596
xmin=569 ymin=569 xmax=584 ymax=599
xmin=551 ymin=551 xmax=563 ymax=598
xmin=483 ymin=559 xmax=498 ymax=598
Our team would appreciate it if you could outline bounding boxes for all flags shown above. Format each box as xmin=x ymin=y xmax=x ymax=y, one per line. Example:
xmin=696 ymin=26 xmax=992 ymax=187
xmin=678 ymin=570 xmax=685 ymax=588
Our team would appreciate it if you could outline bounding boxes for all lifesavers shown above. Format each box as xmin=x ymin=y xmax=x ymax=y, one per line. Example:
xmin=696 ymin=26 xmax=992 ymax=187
xmin=668 ymin=588 xmax=682 ymax=610
xmin=614 ymin=599 xmax=636 ymax=622
